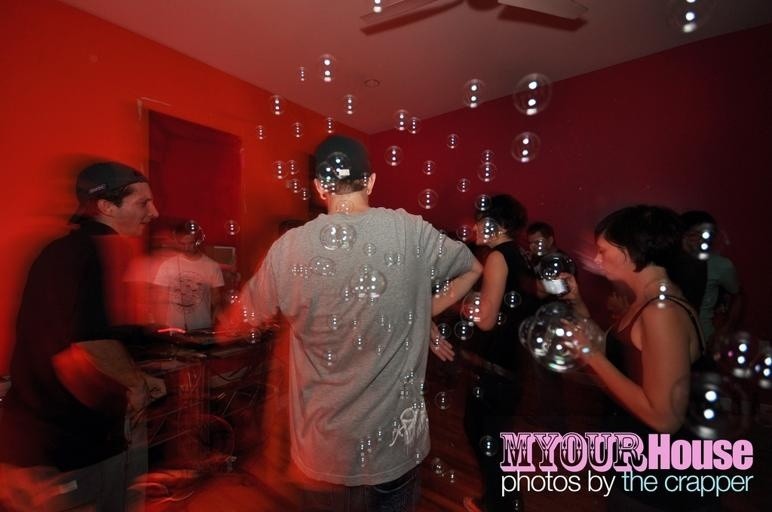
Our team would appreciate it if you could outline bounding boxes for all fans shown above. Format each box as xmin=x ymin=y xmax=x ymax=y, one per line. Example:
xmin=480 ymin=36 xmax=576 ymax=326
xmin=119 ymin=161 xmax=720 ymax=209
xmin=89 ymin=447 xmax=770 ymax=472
xmin=360 ymin=0 xmax=589 ymax=30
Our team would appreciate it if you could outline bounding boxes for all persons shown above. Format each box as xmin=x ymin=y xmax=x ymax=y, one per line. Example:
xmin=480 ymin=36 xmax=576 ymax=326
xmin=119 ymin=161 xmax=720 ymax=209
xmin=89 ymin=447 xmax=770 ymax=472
xmin=213 ymin=135 xmax=483 ymax=512
xmin=152 ymin=222 xmax=224 ymax=331
xmin=555 ymin=203 xmax=721 ymax=511
xmin=664 ymin=209 xmax=745 ymax=372
xmin=10 ymin=161 xmax=167 ymax=511
xmin=451 ymin=192 xmax=539 ymax=511
xmin=521 ymin=221 xmax=573 ymax=436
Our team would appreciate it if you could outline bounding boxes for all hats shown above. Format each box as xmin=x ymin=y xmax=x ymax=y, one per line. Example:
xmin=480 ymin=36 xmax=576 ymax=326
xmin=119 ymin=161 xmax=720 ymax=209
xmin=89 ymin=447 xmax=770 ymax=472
xmin=69 ymin=162 xmax=149 ymax=224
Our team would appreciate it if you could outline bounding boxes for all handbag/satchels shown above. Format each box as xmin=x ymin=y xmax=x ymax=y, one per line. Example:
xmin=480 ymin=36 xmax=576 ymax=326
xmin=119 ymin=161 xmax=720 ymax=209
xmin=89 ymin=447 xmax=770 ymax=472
xmin=114 ymin=322 xmax=183 ymax=363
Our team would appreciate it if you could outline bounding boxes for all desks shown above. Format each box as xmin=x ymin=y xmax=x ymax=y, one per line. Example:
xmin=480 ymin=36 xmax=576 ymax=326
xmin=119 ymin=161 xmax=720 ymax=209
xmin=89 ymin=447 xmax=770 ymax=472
xmin=130 ymin=336 xmax=267 ymax=465
